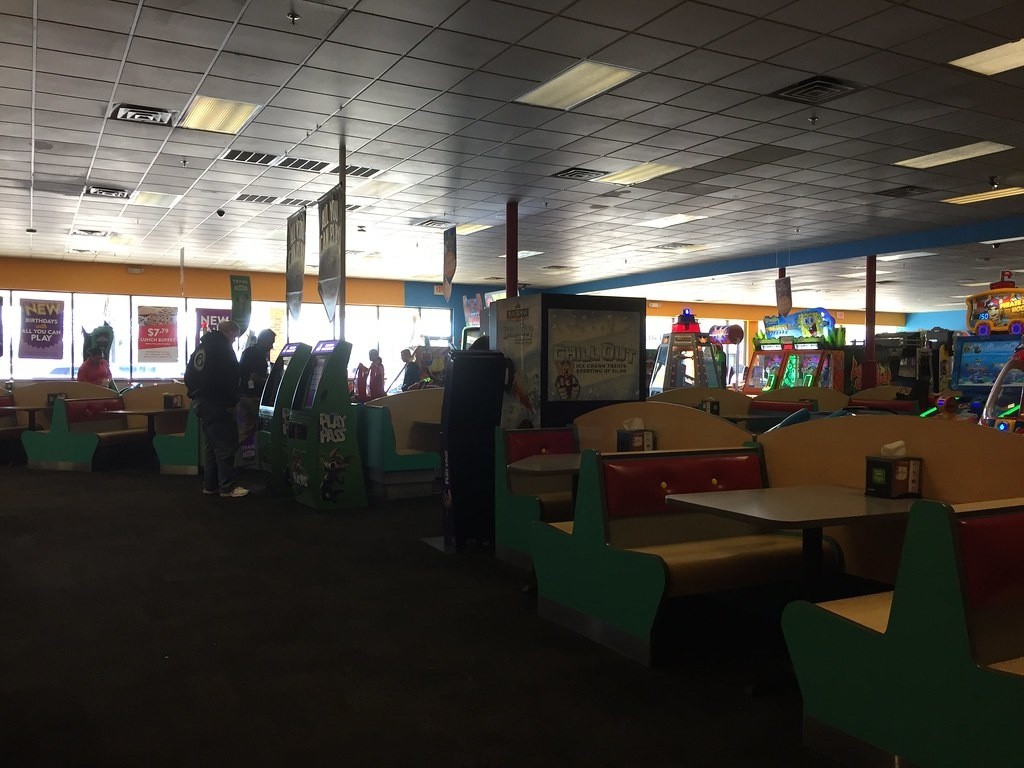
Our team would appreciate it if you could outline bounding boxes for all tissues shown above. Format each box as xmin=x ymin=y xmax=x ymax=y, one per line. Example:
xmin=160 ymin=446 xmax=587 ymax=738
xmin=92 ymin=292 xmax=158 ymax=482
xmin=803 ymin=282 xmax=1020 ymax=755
xmin=865 ymin=439 xmax=924 ymax=500
xmin=616 ymin=416 xmax=656 ymax=452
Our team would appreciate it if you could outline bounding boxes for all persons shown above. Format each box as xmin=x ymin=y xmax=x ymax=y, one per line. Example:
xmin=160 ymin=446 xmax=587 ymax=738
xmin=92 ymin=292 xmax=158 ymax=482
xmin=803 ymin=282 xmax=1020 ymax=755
xmin=75 ymin=347 xmax=111 ymax=388
xmin=238 ymin=328 xmax=276 ymax=436
xmin=368 ymin=349 xmax=381 ymax=363
xmin=401 ymin=349 xmax=419 ymax=392
xmin=182 ymin=320 xmax=249 ymax=498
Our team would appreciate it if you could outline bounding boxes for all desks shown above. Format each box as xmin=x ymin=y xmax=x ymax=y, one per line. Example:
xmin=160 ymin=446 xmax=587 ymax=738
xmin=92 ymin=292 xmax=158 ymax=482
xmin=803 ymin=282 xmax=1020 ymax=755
xmin=101 ymin=409 xmax=177 ymax=465
xmin=0 ymin=406 xmax=54 ymax=447
xmin=664 ymin=484 xmax=959 ymax=666
xmin=416 ymin=416 xmax=444 ymax=427
xmin=504 ymin=452 xmax=583 ymax=526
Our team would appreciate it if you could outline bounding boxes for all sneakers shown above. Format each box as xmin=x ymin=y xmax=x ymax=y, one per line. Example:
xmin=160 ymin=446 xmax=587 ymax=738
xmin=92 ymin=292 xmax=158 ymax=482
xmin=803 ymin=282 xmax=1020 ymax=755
xmin=202 ymin=488 xmax=221 ymax=495
xmin=221 ymin=487 xmax=250 ymax=498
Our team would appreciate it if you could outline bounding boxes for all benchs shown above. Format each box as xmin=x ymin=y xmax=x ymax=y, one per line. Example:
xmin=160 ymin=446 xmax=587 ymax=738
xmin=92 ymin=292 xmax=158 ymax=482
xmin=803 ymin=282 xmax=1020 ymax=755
xmin=365 ymin=403 xmax=441 ymax=505
xmin=0 ymin=396 xmax=34 ymax=440
xmin=21 ymin=396 xmax=59 ymax=473
xmin=777 ymin=495 xmax=1024 ymax=758
xmin=524 ymin=449 xmax=584 ymax=636
xmin=59 ymin=395 xmax=129 ymax=470
xmin=584 ymin=446 xmax=846 ymax=666
xmin=495 ymin=424 xmax=584 ymax=566
xmin=153 ymin=401 xmax=200 ymax=476
xmin=932 ymin=499 xmax=1024 ymax=768
